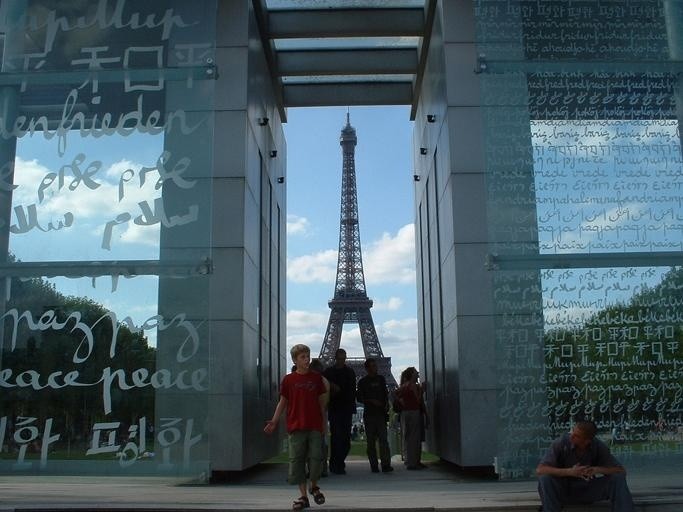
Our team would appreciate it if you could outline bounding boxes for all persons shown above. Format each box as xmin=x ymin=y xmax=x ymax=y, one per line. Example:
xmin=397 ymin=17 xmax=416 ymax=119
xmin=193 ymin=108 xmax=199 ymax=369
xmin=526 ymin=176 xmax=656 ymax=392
xmin=263 ymin=344 xmax=357 ymax=510
xmin=536 ymin=421 xmax=633 ymax=512
xmin=357 ymin=358 xmax=393 ymax=473
xmin=393 ymin=367 xmax=428 ymax=470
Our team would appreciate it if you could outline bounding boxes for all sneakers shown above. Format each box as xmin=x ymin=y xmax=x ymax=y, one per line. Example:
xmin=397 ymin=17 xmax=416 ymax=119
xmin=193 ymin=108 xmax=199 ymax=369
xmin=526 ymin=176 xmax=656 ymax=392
xmin=407 ymin=463 xmax=428 ymax=470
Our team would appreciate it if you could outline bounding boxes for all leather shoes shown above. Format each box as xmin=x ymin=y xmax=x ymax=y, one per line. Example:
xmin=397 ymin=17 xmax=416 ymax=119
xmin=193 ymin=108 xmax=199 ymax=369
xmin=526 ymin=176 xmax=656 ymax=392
xmin=372 ymin=466 xmax=393 ymax=473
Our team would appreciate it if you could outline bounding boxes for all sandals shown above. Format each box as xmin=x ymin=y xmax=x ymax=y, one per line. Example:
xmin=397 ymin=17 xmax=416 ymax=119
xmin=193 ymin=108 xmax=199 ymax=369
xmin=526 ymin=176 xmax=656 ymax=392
xmin=309 ymin=486 xmax=325 ymax=505
xmin=293 ymin=496 xmax=310 ymax=511
